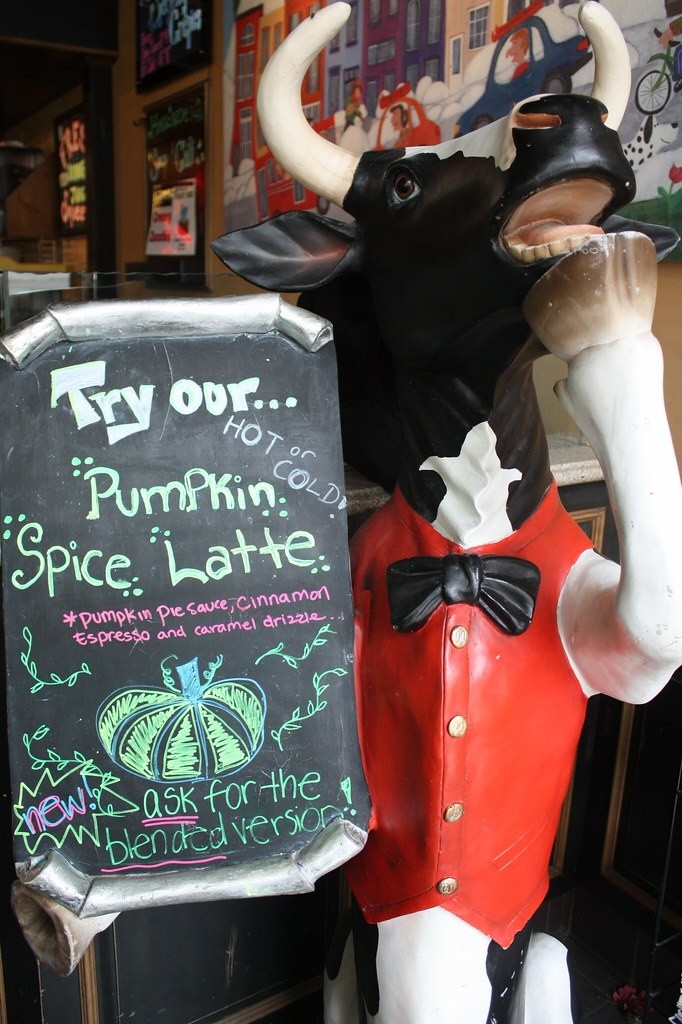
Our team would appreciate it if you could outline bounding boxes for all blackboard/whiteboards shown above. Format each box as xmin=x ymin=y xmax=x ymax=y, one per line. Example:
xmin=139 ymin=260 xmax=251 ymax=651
xmin=0 ymin=290 xmax=374 ymax=921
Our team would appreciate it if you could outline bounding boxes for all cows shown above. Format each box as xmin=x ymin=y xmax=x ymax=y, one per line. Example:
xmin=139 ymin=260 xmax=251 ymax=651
xmin=204 ymin=0 xmax=682 ymax=1023
